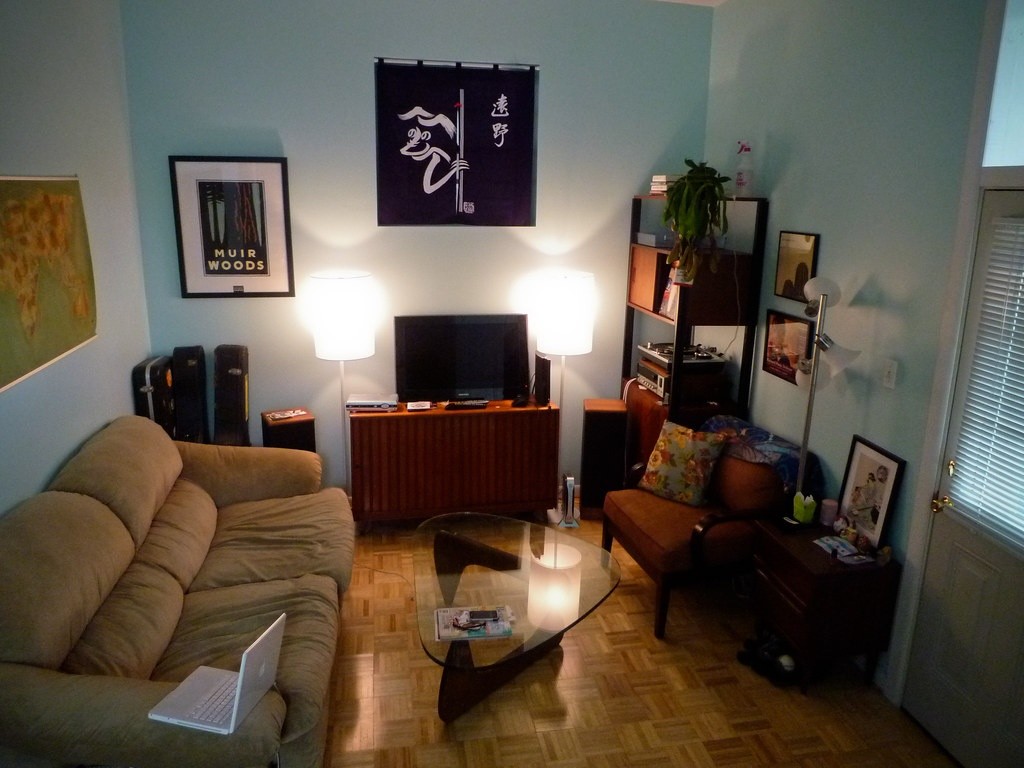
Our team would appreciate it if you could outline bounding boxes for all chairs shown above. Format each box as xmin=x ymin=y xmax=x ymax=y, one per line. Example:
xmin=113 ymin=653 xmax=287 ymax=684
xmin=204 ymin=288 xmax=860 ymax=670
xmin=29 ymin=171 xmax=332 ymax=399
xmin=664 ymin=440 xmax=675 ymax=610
xmin=601 ymin=418 xmax=814 ymax=640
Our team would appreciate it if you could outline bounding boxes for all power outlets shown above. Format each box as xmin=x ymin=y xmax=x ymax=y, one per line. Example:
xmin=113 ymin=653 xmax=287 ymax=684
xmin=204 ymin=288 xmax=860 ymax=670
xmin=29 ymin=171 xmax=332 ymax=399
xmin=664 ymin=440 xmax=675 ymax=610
xmin=882 ymin=358 xmax=900 ymax=391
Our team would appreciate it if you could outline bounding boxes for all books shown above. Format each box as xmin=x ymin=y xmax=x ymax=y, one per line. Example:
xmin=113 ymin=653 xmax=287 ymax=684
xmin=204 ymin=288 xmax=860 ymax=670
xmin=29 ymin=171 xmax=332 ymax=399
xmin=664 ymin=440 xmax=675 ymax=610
xmin=433 ymin=604 xmax=513 ymax=642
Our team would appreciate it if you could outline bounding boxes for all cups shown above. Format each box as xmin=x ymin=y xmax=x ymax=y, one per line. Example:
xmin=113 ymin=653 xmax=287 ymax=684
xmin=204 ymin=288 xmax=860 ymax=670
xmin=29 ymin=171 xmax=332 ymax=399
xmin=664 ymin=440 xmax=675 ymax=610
xmin=821 ymin=498 xmax=838 ymax=526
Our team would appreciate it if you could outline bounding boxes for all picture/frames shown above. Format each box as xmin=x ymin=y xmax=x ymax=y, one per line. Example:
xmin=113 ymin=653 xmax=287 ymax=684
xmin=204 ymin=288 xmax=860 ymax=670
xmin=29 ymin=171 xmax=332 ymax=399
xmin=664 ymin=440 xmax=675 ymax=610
xmin=761 ymin=311 xmax=813 ymax=384
xmin=832 ymin=435 xmax=905 ymax=544
xmin=169 ymin=154 xmax=296 ymax=298
xmin=775 ymin=231 xmax=820 ymax=303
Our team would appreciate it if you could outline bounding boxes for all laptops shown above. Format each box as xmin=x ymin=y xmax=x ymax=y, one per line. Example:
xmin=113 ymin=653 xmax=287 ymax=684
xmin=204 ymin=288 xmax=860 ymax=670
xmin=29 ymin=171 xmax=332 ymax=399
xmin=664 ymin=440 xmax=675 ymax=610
xmin=148 ymin=613 xmax=287 ymax=735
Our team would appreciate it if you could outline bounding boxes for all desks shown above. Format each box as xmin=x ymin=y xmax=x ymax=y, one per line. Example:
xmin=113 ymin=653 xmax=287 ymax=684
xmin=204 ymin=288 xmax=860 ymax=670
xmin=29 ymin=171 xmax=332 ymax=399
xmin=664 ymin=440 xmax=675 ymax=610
xmin=352 ymin=395 xmax=561 ymax=537
xmin=262 ymin=407 xmax=317 ymax=448
xmin=736 ymin=514 xmax=902 ymax=689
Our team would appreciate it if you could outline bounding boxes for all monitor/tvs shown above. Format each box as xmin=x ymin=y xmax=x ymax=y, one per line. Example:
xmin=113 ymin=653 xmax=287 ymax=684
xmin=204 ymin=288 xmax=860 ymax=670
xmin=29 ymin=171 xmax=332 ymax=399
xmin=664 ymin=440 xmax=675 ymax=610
xmin=394 ymin=314 xmax=530 ymax=404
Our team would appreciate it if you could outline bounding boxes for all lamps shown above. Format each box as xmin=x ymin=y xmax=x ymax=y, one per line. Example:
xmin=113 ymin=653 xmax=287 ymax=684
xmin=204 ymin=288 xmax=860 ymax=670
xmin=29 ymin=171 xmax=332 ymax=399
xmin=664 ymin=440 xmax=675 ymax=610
xmin=311 ymin=269 xmax=380 ymax=499
xmin=537 ymin=270 xmax=597 ymax=523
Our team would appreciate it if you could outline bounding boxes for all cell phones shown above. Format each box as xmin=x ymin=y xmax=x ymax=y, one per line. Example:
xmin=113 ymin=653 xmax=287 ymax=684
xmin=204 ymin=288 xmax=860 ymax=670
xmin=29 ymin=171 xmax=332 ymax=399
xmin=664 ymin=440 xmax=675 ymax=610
xmin=469 ymin=610 xmax=499 ymax=621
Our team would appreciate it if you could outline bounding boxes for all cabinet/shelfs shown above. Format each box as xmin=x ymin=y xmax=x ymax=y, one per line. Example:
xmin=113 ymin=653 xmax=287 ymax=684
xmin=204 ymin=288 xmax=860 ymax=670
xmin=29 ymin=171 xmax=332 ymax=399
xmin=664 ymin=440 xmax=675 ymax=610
xmin=621 ymin=192 xmax=770 ymax=472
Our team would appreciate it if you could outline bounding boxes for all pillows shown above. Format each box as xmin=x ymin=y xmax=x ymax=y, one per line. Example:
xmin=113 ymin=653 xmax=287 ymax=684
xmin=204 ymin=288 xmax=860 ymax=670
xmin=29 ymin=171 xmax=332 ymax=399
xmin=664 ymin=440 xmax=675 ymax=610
xmin=637 ymin=421 xmax=735 ymax=504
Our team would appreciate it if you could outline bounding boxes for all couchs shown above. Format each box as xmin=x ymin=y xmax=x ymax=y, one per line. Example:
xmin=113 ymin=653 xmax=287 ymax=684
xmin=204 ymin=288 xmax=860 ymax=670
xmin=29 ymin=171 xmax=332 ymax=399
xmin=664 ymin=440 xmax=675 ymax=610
xmin=0 ymin=413 xmax=359 ymax=768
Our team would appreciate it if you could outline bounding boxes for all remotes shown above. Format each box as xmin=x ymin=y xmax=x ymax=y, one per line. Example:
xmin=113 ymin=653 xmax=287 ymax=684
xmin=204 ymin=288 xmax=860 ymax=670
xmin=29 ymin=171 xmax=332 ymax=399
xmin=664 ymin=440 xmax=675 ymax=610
xmin=444 ymin=400 xmax=489 ymax=410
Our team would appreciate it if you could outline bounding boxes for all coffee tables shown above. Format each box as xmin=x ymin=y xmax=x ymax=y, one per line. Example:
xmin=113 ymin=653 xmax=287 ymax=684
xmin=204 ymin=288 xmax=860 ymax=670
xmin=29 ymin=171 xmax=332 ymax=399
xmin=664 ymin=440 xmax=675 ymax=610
xmin=412 ymin=512 xmax=622 ymax=721
